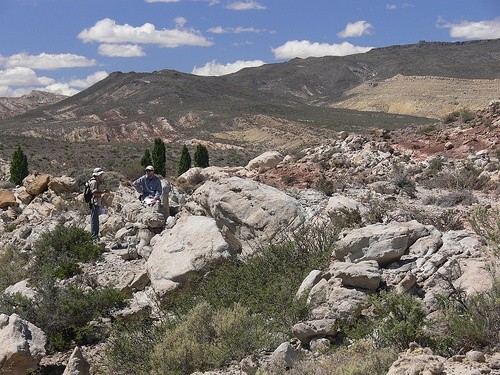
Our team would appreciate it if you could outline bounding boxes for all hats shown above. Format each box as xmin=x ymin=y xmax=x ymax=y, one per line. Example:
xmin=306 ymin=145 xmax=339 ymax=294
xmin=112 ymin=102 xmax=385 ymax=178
xmin=92 ymin=168 xmax=105 ymax=176
xmin=146 ymin=165 xmax=154 ymax=170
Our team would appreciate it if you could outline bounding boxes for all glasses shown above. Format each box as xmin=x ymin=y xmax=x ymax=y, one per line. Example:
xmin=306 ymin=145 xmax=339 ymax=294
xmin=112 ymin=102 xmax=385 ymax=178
xmin=146 ymin=170 xmax=153 ymax=172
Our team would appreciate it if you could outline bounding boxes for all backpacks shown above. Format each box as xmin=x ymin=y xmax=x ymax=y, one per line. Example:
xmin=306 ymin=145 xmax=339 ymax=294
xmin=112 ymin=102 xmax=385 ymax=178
xmin=83 ymin=179 xmax=97 ymax=203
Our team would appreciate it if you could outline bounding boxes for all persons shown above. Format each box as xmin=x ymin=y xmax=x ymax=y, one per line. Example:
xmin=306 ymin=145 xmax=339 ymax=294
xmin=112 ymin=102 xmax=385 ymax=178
xmin=89 ymin=168 xmax=108 ymax=240
xmin=132 ymin=166 xmax=162 ymax=206
